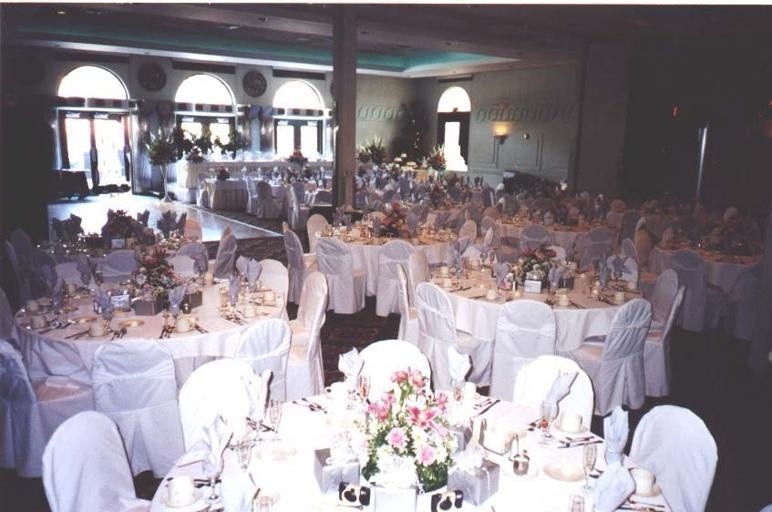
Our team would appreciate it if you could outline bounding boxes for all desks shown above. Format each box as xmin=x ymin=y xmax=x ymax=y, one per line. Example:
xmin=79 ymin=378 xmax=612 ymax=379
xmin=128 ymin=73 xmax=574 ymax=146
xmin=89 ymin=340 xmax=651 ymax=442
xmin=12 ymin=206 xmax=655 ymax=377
xmin=152 ymin=387 xmax=669 ymax=512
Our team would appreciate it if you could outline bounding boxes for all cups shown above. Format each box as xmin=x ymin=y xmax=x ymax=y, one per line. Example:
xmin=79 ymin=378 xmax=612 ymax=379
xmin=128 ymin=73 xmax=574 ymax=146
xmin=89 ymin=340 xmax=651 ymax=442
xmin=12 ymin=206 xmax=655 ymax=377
xmin=17 ymin=267 xmax=274 ymax=343
xmin=162 ymin=378 xmax=669 ymax=511
xmin=435 ymin=248 xmax=642 ymax=309
xmin=321 ymin=223 xmax=387 ymax=246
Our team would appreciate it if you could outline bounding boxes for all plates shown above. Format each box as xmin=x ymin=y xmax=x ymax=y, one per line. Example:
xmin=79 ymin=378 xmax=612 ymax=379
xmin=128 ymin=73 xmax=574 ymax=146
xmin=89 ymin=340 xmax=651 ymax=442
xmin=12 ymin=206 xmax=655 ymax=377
xmin=703 ymin=254 xmax=722 ymax=261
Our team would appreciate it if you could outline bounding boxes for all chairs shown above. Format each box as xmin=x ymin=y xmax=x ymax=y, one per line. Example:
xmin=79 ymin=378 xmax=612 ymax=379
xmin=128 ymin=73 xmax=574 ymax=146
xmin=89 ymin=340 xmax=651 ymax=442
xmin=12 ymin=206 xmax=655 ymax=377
xmin=179 ymin=358 xmax=260 ymax=453
xmin=27 ymin=409 xmax=151 ymax=512
xmin=233 ymin=323 xmax=293 ymax=407
xmin=292 ymin=269 xmax=329 ymax=393
xmin=1 ymin=126 xmax=772 ymax=364
xmin=0 ymin=126 xmax=772 ymax=512
xmin=94 ymin=339 xmax=185 ymax=473
xmin=511 ymin=352 xmax=595 ymax=431
xmin=594 ymin=301 xmax=647 ymax=413
xmin=349 ymin=338 xmax=434 ymax=395
xmin=487 ymin=300 xmax=556 ymax=401
xmin=414 ymin=285 xmax=485 ymax=373
xmin=0 ymin=340 xmax=89 ymax=478
xmin=627 ymin=403 xmax=720 ymax=510
